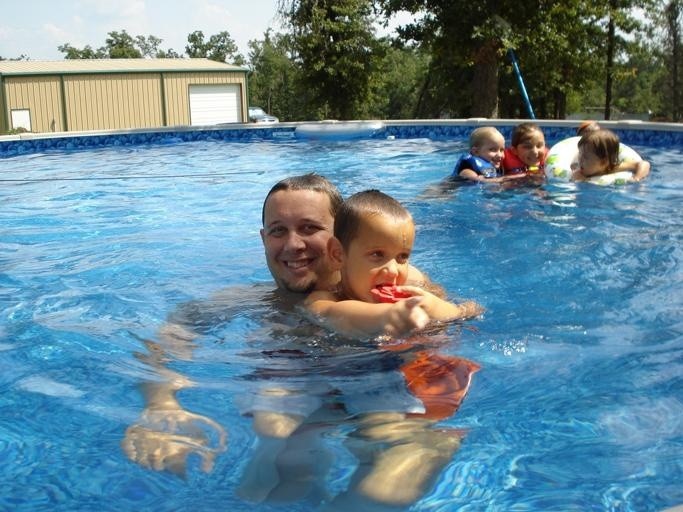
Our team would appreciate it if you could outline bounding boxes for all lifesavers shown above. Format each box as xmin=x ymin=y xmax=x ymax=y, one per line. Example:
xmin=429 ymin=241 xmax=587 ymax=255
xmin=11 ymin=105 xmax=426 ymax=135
xmin=545 ymin=136 xmax=643 ymax=188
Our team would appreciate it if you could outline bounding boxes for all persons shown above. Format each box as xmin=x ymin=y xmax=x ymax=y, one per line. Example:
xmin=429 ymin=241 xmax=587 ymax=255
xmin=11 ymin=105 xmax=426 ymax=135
xmin=116 ymin=168 xmax=347 ymax=503
xmin=262 ymin=189 xmax=486 ymax=508
xmin=406 ymin=125 xmax=527 ymax=201
xmin=569 ymin=122 xmax=650 ymax=186
xmin=499 ymin=121 xmax=549 ymax=189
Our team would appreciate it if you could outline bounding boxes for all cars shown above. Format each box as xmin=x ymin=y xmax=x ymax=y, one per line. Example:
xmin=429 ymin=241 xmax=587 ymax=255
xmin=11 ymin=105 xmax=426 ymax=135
xmin=248 ymin=106 xmax=278 ymax=123
